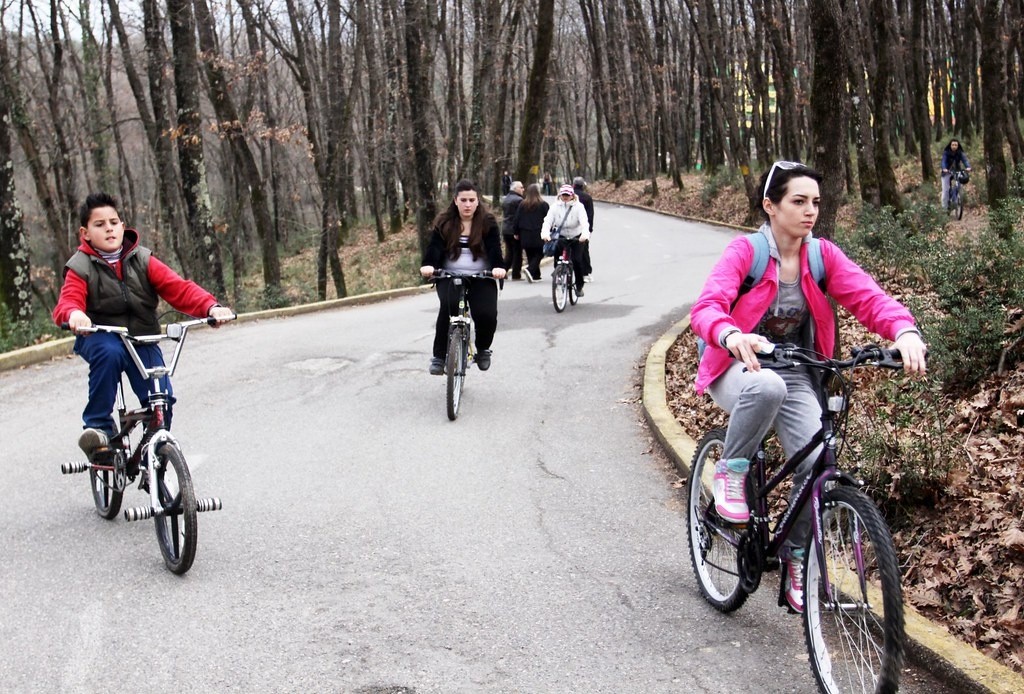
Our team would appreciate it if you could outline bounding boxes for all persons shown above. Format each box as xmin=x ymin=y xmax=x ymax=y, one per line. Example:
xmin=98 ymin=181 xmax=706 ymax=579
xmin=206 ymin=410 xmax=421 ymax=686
xmin=690 ymin=161 xmax=925 ymax=612
xmin=502 ymin=168 xmax=594 ymax=298
xmin=940 ymin=139 xmax=972 ymax=212
xmin=52 ymin=193 xmax=234 ymax=493
xmin=419 ymin=179 xmax=506 ymax=376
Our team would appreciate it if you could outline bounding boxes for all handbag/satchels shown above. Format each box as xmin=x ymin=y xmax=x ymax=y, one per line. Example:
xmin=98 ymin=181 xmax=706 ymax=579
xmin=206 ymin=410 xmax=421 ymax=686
xmin=542 ymin=227 xmax=560 ymax=257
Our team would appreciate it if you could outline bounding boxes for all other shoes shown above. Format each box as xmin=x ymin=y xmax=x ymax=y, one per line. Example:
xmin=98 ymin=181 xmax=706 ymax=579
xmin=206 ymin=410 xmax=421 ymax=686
xmin=79 ymin=428 xmax=111 ymax=464
xmin=576 ymin=288 xmax=584 ymax=296
xmin=524 ymin=268 xmax=542 ymax=282
xmin=477 ymin=348 xmax=491 ymax=370
xmin=430 ymin=357 xmax=445 ymax=375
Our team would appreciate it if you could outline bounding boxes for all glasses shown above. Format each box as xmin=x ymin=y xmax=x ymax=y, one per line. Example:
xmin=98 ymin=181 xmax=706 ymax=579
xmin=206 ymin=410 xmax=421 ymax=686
xmin=763 ymin=160 xmax=808 ymax=199
xmin=563 ymin=194 xmax=570 ymax=196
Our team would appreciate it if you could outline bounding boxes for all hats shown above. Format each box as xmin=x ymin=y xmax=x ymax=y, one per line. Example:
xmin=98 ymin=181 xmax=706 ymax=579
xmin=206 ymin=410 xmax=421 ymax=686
xmin=559 ymin=183 xmax=574 ymax=195
xmin=574 ymin=176 xmax=584 ymax=185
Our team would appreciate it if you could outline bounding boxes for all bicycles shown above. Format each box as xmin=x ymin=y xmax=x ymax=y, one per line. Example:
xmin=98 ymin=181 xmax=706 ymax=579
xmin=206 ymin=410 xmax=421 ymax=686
xmin=684 ymin=342 xmax=930 ymax=694
xmin=547 ymin=237 xmax=589 ymax=312
xmin=423 ymin=269 xmax=506 ymax=421
xmin=60 ymin=311 xmax=238 ymax=576
xmin=942 ymin=171 xmax=970 ymax=220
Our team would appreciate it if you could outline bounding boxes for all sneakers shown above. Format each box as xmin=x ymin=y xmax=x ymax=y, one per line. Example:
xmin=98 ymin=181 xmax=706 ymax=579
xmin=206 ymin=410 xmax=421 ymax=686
xmin=774 ymin=532 xmax=804 ymax=614
xmin=713 ymin=458 xmax=750 ymax=524
